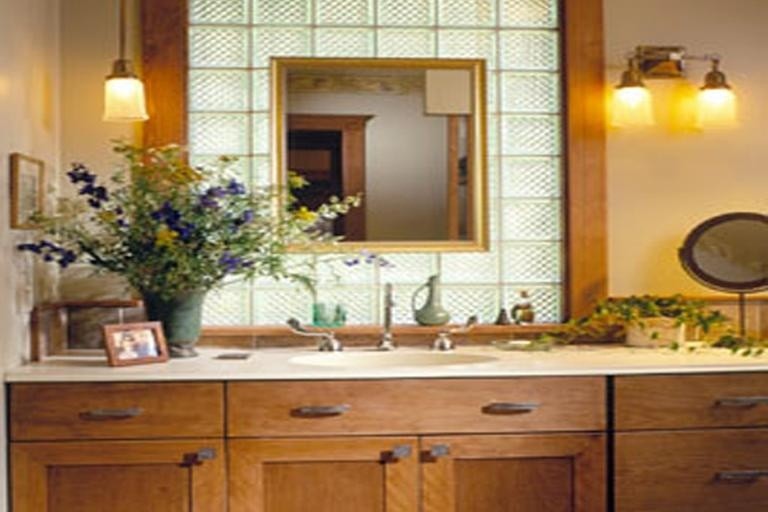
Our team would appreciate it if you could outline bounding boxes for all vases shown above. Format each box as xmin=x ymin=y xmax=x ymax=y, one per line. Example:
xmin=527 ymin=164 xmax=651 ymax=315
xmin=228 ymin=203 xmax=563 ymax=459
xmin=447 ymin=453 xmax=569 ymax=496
xmin=623 ymin=316 xmax=686 ymax=346
xmin=135 ymin=289 xmax=207 ymax=345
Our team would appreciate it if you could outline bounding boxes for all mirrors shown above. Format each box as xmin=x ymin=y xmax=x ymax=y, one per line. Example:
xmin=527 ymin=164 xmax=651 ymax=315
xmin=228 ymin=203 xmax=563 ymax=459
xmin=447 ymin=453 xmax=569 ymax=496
xmin=678 ymin=214 xmax=768 ymax=343
xmin=265 ymin=53 xmax=491 ymax=255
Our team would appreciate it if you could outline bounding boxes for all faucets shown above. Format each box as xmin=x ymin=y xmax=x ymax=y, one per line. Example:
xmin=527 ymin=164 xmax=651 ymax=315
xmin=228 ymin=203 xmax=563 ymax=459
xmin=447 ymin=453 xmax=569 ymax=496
xmin=384 ymin=283 xmax=395 ymax=335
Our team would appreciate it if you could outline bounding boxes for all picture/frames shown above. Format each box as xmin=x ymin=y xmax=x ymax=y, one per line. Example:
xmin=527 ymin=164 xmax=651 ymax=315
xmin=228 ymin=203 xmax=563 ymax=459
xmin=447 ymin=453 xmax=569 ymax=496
xmin=100 ymin=320 xmax=173 ymax=367
xmin=5 ymin=150 xmax=48 ymax=233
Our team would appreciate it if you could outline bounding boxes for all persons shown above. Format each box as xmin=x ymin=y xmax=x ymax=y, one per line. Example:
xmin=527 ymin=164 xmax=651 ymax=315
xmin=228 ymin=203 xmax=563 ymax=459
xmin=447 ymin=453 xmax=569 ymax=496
xmin=117 ymin=330 xmax=150 ymax=359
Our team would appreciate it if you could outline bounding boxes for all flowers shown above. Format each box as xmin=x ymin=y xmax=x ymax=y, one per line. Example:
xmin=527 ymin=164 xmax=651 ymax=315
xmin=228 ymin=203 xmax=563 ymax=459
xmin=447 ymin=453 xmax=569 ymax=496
xmin=519 ymin=291 xmax=766 ymax=364
xmin=16 ymin=136 xmax=374 ymax=297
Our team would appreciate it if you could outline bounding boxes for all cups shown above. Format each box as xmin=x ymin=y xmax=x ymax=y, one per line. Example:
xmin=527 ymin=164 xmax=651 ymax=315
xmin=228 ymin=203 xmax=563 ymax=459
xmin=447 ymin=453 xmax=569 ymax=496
xmin=313 ymin=284 xmax=348 ymax=326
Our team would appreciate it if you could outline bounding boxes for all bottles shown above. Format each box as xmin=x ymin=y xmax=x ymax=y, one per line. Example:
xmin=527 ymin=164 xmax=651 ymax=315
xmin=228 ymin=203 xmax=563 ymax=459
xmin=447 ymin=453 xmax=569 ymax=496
xmin=412 ymin=272 xmax=452 ymax=325
xmin=513 ymin=288 xmax=534 ymax=326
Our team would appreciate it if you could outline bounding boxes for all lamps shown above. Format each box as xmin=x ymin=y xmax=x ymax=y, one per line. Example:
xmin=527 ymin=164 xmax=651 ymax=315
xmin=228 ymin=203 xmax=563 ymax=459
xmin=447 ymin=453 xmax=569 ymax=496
xmin=100 ymin=1 xmax=149 ymax=125
xmin=612 ymin=46 xmax=734 ymax=106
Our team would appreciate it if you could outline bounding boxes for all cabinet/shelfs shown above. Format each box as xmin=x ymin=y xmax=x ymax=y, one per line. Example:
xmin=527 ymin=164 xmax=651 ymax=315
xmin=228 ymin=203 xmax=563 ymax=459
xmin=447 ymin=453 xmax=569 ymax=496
xmin=228 ymin=378 xmax=612 ymax=510
xmin=286 ymin=112 xmax=375 ymax=242
xmin=7 ymin=381 xmax=226 ymax=511
xmin=612 ymin=373 xmax=766 ymax=511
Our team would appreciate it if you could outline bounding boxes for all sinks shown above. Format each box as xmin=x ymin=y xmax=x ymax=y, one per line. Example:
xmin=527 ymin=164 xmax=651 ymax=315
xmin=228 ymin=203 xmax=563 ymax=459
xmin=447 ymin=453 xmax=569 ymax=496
xmin=288 ymin=352 xmax=500 ymax=367
